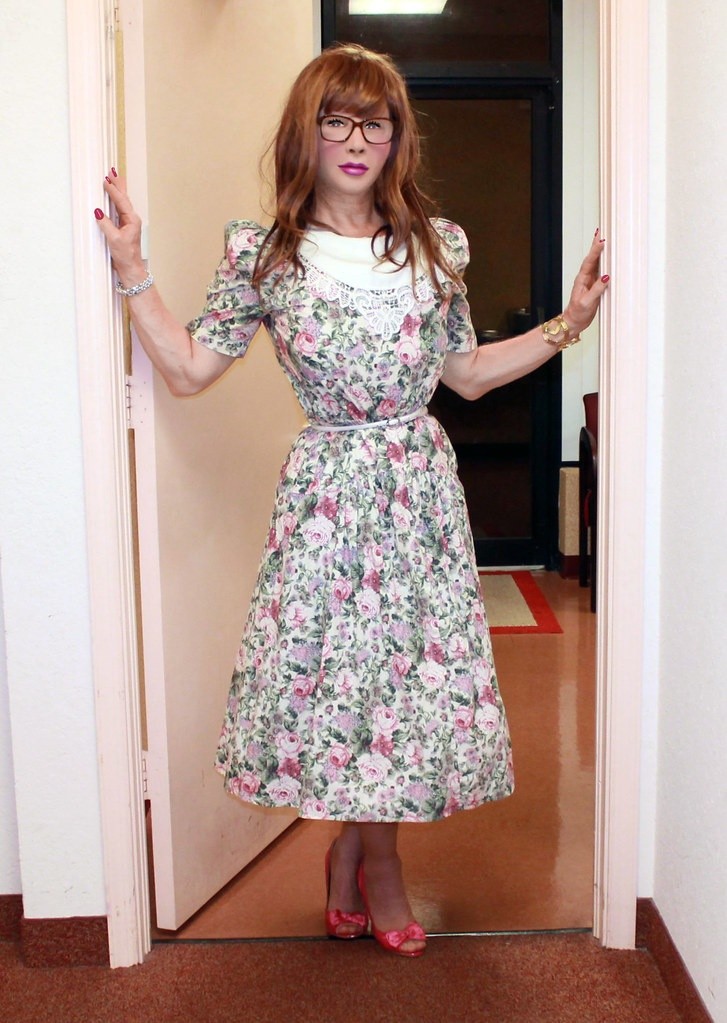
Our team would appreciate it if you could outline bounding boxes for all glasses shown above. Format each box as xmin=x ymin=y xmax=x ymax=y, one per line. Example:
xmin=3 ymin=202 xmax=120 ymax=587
xmin=317 ymin=113 xmax=398 ymax=144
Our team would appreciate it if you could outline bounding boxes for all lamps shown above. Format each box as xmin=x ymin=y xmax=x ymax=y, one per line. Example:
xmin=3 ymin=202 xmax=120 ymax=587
xmin=334 ymin=0 xmax=456 ymax=23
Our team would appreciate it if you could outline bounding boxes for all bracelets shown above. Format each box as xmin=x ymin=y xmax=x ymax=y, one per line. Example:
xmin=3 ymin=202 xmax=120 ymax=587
xmin=541 ymin=312 xmax=582 ymax=352
xmin=113 ymin=269 xmax=155 ymax=296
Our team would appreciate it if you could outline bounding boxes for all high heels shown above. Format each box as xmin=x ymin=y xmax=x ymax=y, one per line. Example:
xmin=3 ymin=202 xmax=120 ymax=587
xmin=358 ymin=858 xmax=427 ymax=956
xmin=324 ymin=836 xmax=368 ymax=938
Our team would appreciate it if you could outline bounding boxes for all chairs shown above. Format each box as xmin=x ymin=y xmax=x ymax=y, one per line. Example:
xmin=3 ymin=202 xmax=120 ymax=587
xmin=581 ymin=389 xmax=602 ymax=608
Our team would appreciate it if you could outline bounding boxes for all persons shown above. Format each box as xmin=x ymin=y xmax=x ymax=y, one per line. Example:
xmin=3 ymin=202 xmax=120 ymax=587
xmin=95 ymin=38 xmax=613 ymax=960
